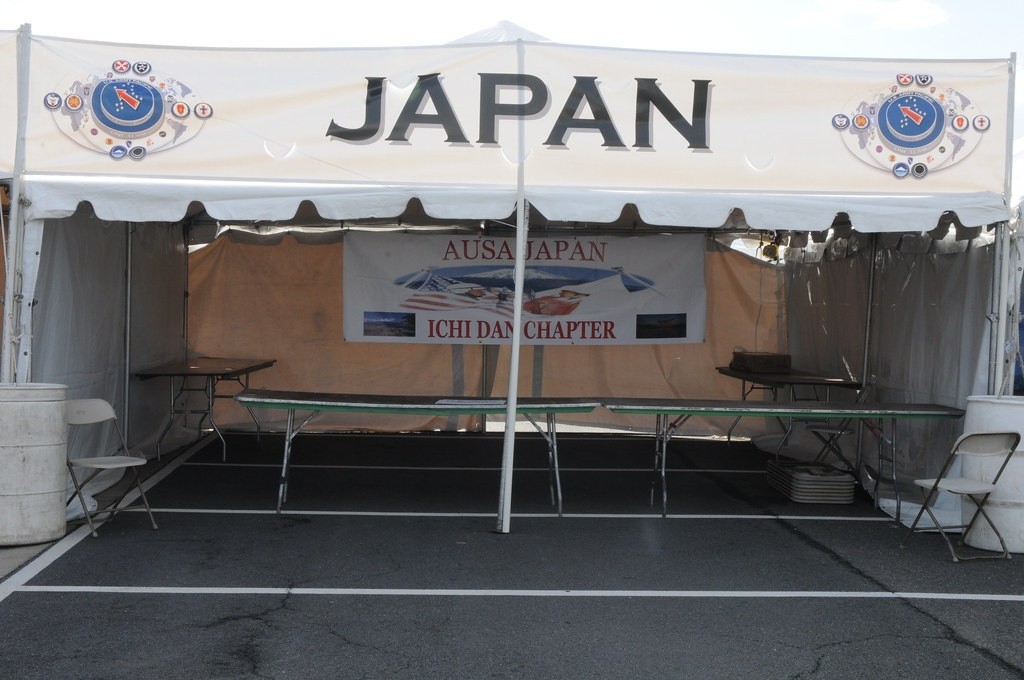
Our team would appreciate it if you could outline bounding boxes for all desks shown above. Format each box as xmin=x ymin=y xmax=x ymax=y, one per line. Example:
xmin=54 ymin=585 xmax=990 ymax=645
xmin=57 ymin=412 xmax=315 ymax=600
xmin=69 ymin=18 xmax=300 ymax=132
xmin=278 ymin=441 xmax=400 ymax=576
xmin=136 ymin=356 xmax=276 ymax=462
xmin=719 ymin=367 xmax=869 ymax=474
xmin=236 ymin=388 xmax=600 ymax=518
xmin=596 ymin=397 xmax=965 ymax=527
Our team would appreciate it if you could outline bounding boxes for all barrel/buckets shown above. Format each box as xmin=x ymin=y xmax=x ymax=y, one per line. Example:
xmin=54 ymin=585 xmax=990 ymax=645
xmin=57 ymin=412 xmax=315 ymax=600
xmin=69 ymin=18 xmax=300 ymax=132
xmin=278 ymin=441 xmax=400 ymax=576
xmin=0 ymin=382 xmax=69 ymax=547
xmin=960 ymin=395 xmax=1024 ymax=555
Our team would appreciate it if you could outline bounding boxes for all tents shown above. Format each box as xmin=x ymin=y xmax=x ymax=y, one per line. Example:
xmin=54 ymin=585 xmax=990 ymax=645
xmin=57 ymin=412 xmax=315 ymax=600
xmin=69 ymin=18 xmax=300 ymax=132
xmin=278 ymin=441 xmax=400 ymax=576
xmin=1 ymin=22 xmax=1019 ymax=537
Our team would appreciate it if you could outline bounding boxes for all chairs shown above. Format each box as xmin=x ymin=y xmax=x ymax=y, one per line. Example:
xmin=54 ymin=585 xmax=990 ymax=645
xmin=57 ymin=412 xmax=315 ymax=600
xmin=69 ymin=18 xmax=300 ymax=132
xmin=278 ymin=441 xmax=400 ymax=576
xmin=900 ymin=429 xmax=1021 ymax=563
xmin=64 ymin=398 xmax=157 ymax=537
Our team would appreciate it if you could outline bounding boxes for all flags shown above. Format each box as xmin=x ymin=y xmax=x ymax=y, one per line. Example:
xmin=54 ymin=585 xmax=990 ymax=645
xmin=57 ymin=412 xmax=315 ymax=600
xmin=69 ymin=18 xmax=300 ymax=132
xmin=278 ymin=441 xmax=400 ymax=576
xmin=397 ymin=272 xmax=514 ymax=320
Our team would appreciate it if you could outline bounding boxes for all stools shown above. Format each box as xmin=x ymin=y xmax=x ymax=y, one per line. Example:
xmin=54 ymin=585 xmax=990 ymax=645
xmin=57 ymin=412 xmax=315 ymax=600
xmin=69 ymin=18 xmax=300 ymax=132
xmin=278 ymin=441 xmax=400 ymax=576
xmin=806 ymin=425 xmax=858 ymax=478
